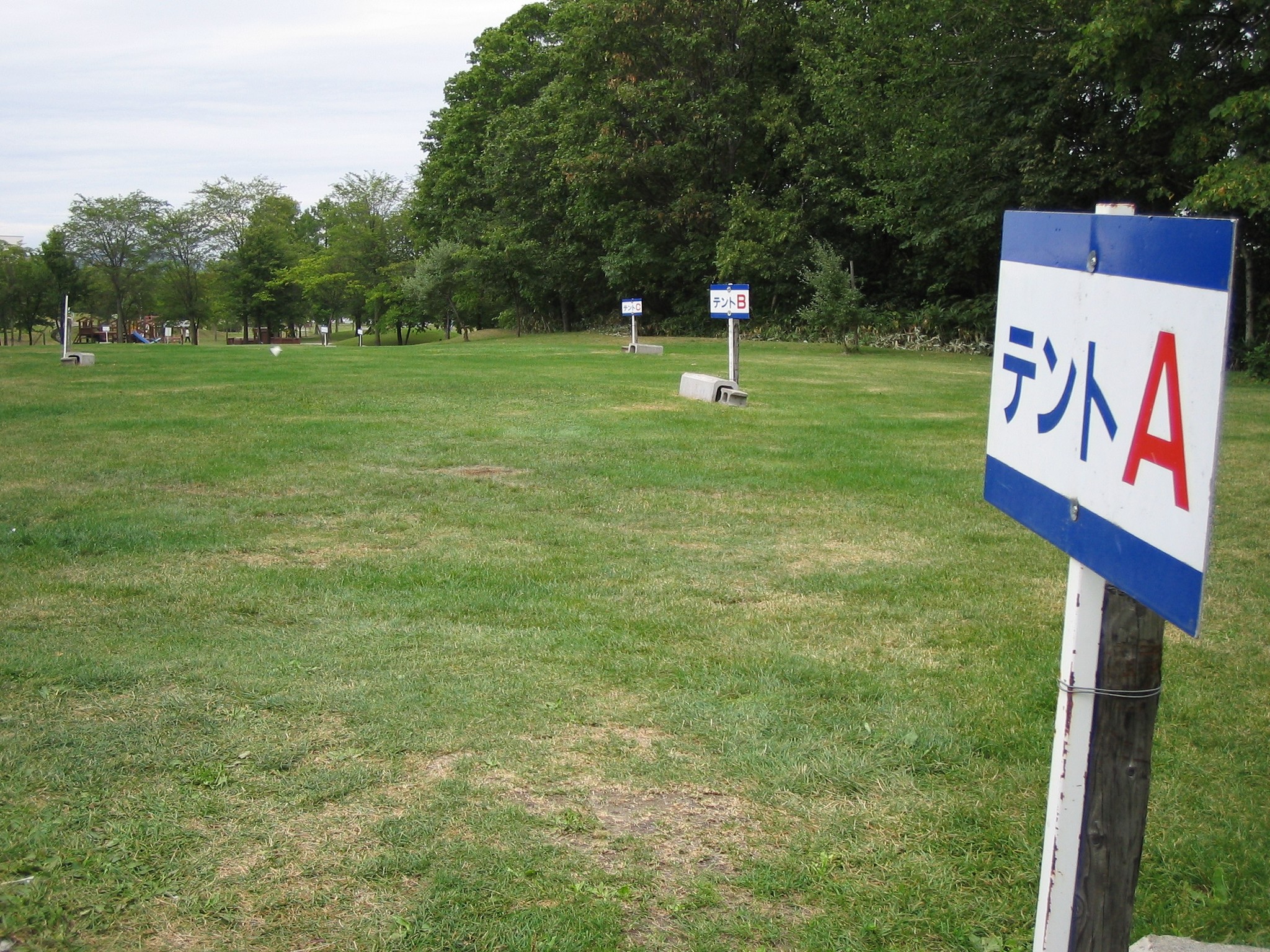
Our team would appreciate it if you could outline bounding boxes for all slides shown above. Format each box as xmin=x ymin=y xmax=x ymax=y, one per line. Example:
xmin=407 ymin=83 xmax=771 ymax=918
xmin=132 ymin=330 xmax=150 ymax=344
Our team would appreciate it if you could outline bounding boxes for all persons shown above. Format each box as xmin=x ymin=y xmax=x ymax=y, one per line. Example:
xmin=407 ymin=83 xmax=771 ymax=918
xmin=185 ymin=327 xmax=191 ymax=342
